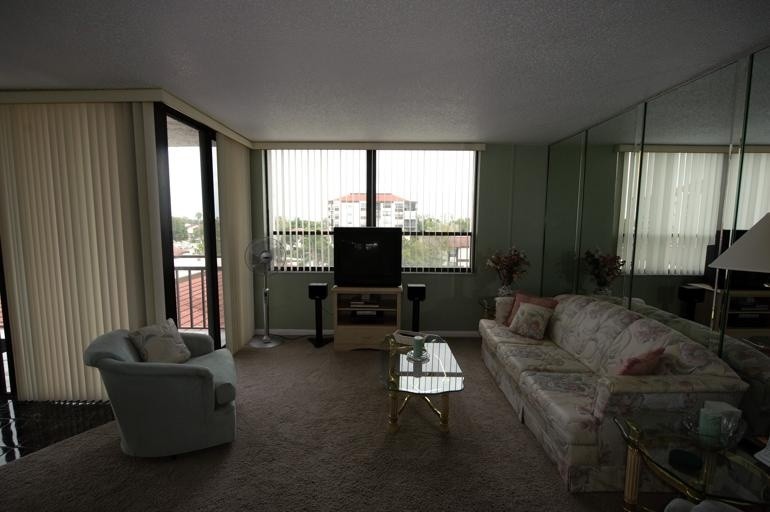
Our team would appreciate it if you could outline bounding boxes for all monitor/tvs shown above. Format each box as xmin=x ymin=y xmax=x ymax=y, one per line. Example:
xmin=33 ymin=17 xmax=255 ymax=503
xmin=703 ymin=228 xmax=768 ymax=292
xmin=333 ymin=226 xmax=402 ymax=288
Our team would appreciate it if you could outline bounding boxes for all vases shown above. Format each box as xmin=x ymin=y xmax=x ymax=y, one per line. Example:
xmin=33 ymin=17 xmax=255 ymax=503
xmin=498 ymin=285 xmax=515 ymax=297
xmin=590 ymin=287 xmax=612 ymax=296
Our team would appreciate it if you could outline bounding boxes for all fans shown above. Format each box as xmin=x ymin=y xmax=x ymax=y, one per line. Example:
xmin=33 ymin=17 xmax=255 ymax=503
xmin=245 ymin=239 xmax=286 ymax=349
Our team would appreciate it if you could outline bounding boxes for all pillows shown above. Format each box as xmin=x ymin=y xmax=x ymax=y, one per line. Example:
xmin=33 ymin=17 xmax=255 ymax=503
xmin=130 ymin=318 xmax=189 ymax=364
xmin=616 ymin=347 xmax=666 ymax=375
xmin=508 ymin=302 xmax=552 ymax=339
xmin=504 ymin=293 xmax=558 ymax=329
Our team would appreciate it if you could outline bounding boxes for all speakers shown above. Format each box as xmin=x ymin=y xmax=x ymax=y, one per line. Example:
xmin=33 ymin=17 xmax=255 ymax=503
xmin=407 ymin=283 xmax=427 ymax=301
xmin=308 ymin=282 xmax=328 ymax=299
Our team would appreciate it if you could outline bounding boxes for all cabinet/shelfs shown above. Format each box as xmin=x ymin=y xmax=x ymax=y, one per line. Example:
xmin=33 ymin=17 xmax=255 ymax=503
xmin=330 ymin=286 xmax=402 ymax=352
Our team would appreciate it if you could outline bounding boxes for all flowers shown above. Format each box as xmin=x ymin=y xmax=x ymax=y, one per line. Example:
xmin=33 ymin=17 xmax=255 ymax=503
xmin=573 ymin=248 xmax=625 ymax=286
xmin=485 ymin=247 xmax=531 ymax=286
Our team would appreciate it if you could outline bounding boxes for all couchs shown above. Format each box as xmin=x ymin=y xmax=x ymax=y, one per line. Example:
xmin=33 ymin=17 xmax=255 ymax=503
xmin=83 ymin=332 xmax=239 ymax=458
xmin=619 ymin=295 xmax=769 ymax=435
xmin=479 ymin=295 xmax=746 ymax=500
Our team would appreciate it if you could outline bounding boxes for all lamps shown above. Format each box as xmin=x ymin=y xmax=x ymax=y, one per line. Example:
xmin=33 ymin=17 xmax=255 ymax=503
xmin=711 ymin=207 xmax=768 ymax=466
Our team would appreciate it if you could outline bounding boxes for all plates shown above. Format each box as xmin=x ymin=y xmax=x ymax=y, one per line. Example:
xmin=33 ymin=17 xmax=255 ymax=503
xmin=407 ymin=350 xmax=430 ymax=362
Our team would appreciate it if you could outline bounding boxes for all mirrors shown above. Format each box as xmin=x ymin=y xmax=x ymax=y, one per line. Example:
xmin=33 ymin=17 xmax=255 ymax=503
xmin=628 ymin=51 xmax=752 ymax=360
xmin=719 ymin=41 xmax=770 ymax=422
xmin=585 ymin=100 xmax=644 ymax=314
xmin=541 ymin=130 xmax=584 ymax=295
